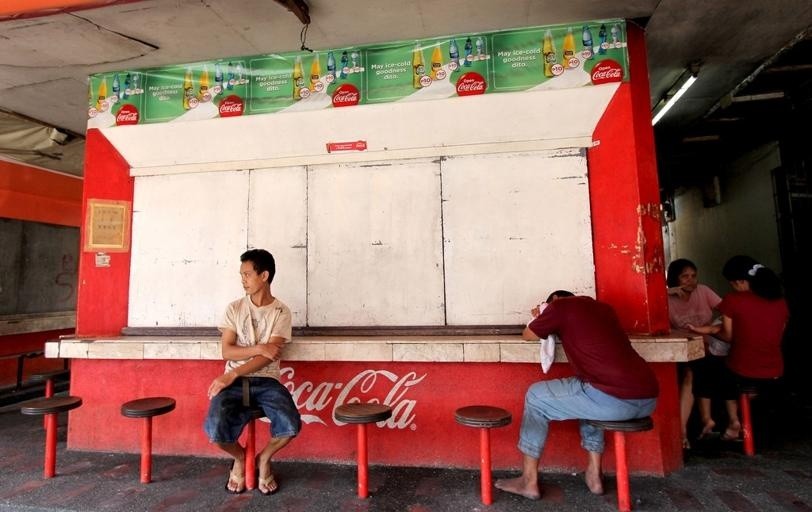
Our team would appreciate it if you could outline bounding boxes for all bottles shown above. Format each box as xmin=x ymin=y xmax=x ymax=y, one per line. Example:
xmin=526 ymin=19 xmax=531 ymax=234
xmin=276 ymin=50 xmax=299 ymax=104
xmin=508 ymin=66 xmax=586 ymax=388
xmin=88 ymin=74 xmax=140 ymax=118
xmin=544 ymin=25 xmax=622 ymax=78
xmin=181 ymin=61 xmax=245 ymax=112
xmin=294 ymin=52 xmax=360 ymax=100
xmin=410 ymin=38 xmax=483 ymax=90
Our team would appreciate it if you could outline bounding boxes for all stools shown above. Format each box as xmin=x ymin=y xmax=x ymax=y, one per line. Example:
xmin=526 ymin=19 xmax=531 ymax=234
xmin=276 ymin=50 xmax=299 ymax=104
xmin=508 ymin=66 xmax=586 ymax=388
xmin=232 ymin=401 xmax=270 ymax=491
xmin=721 ymin=379 xmax=765 ymax=458
xmin=450 ymin=403 xmax=514 ymax=506
xmin=330 ymin=399 xmax=393 ymax=498
xmin=24 ymin=367 xmax=70 ymax=427
xmin=581 ymin=411 xmax=658 ymax=511
xmin=118 ymin=393 xmax=178 ymax=485
xmin=20 ymin=394 xmax=86 ymax=479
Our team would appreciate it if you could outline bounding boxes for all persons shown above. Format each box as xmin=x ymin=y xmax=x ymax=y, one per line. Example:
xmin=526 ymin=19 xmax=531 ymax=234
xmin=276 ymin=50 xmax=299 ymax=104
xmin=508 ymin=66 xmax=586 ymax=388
xmin=205 ymin=250 xmax=304 ymax=494
xmin=664 ymin=257 xmax=786 ymax=451
xmin=494 ymin=291 xmax=659 ymax=500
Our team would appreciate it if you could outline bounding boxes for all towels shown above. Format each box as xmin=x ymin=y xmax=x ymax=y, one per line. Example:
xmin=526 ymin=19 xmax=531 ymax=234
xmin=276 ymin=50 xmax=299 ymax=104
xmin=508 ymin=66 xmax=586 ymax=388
xmin=526 ymin=301 xmax=558 ymax=375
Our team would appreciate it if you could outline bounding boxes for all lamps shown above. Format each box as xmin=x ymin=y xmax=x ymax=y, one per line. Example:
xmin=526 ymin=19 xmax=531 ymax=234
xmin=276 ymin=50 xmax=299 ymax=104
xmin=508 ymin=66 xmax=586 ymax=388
xmin=651 ymin=63 xmax=700 ymax=132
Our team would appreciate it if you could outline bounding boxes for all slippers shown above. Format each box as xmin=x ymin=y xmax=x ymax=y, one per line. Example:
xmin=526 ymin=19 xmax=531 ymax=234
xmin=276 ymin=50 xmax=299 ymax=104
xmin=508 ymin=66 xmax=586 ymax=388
xmin=225 ymin=456 xmax=249 ymax=495
xmin=253 ymin=453 xmax=280 ymax=496
